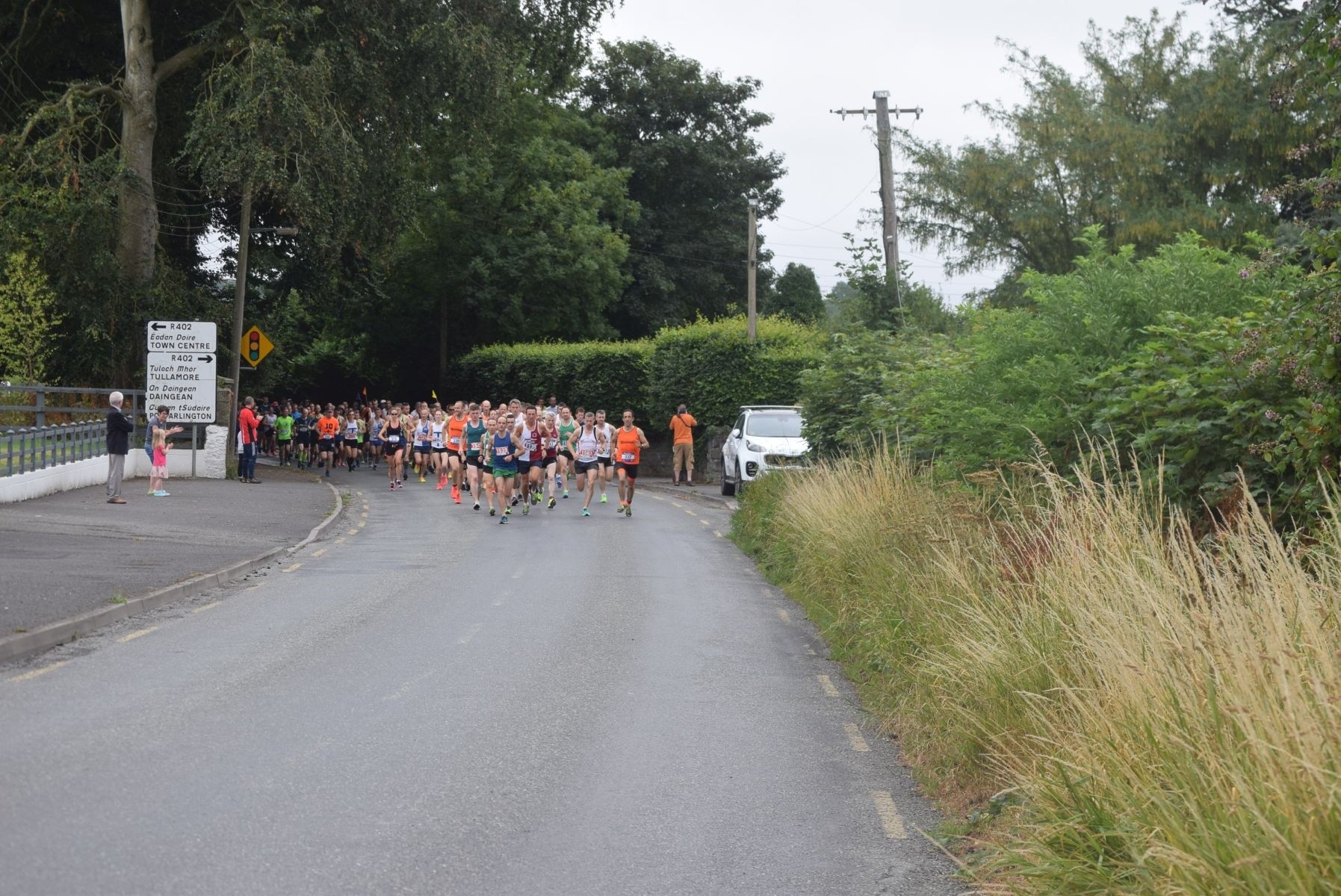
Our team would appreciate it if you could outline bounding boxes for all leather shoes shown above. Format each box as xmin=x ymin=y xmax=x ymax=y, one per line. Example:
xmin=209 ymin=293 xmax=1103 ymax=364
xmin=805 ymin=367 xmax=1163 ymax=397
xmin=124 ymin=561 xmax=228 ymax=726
xmin=107 ymin=498 xmax=126 ymax=503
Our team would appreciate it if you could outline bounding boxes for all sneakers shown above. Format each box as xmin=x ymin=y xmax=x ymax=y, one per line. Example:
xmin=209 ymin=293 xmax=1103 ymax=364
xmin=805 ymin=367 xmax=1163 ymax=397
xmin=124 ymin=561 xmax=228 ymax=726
xmin=242 ymin=478 xmax=247 ymax=482
xmin=147 ymin=491 xmax=154 ymax=496
xmin=257 ymin=447 xmax=632 ymax=525
xmin=162 ymin=490 xmax=170 ymax=495
xmin=246 ymin=478 xmax=262 ymax=483
xmin=154 ymin=492 xmax=170 ymax=496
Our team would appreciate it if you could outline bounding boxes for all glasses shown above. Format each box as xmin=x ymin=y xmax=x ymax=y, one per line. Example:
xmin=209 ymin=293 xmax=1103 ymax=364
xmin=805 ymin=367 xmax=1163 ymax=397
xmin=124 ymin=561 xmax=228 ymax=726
xmin=391 ymin=414 xmax=398 ymax=415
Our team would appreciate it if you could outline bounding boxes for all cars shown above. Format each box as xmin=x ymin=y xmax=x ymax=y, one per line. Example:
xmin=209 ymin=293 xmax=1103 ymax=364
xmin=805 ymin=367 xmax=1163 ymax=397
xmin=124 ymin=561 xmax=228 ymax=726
xmin=716 ymin=405 xmax=818 ymax=499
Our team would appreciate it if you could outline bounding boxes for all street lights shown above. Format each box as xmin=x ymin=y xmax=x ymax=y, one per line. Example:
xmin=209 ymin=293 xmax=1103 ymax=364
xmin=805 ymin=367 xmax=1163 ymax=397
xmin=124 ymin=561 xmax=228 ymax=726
xmin=226 ymin=176 xmax=302 ymax=469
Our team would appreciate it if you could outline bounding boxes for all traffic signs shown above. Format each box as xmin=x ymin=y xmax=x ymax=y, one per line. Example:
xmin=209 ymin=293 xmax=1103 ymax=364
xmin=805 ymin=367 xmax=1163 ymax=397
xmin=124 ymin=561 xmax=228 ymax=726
xmin=146 ymin=321 xmax=216 ymax=424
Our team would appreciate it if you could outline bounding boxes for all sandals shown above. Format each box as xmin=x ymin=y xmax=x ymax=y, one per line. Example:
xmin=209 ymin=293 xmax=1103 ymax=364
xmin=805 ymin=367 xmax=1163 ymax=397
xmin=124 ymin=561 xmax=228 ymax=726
xmin=674 ymin=482 xmax=680 ymax=486
xmin=686 ymin=481 xmax=695 ymax=486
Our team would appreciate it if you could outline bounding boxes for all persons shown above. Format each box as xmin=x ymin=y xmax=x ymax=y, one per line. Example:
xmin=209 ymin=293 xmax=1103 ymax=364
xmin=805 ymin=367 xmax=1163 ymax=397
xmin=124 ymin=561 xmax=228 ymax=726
xmin=610 ymin=409 xmax=649 ymax=517
xmin=537 ymin=411 xmax=556 ymax=508
xmin=556 ymin=407 xmax=580 ymax=498
xmin=535 ymin=410 xmax=545 ymax=425
xmin=669 ymin=404 xmax=697 ymax=487
xmin=233 ymin=393 xmax=546 ymax=524
xmin=545 ymin=396 xmax=557 ymax=415
xmin=144 ymin=405 xmax=184 ymax=495
xmin=574 ymin=408 xmax=586 ymax=426
xmin=555 ymin=401 xmax=573 ymax=489
xmin=594 ymin=410 xmax=616 ymax=502
xmin=151 ymin=429 xmax=171 ymax=496
xmin=514 ymin=406 xmax=551 ymax=514
xmin=106 ymin=391 xmax=134 ymax=504
xmin=567 ymin=412 xmax=606 ymax=516
